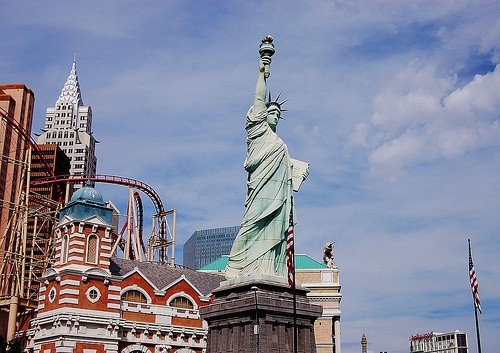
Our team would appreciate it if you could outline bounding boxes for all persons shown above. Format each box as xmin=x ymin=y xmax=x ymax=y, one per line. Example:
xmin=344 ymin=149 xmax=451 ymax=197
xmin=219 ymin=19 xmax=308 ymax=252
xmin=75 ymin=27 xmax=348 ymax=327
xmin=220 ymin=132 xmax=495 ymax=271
xmin=223 ymin=34 xmax=312 ymax=282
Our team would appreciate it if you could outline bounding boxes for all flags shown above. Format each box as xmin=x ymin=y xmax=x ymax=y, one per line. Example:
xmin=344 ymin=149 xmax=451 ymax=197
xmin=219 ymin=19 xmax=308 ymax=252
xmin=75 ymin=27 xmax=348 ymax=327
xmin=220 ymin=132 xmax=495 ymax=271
xmin=284 ymin=207 xmax=294 ymax=288
xmin=468 ymin=246 xmax=482 ymax=314
